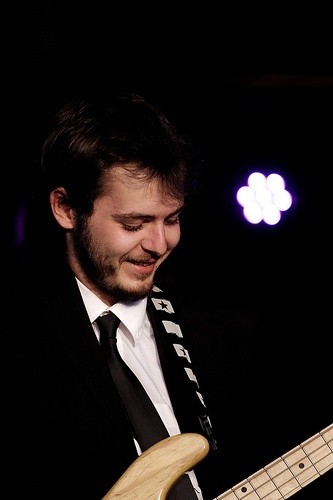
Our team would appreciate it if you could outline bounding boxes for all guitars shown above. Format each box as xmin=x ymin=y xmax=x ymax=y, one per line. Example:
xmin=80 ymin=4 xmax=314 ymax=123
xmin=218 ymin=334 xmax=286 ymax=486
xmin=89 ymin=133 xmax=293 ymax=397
xmin=101 ymin=422 xmax=333 ymax=500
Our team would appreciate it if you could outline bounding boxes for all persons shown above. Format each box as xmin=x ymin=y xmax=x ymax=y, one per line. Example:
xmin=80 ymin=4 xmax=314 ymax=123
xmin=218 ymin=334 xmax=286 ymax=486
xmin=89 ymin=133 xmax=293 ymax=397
xmin=0 ymin=92 xmax=238 ymax=499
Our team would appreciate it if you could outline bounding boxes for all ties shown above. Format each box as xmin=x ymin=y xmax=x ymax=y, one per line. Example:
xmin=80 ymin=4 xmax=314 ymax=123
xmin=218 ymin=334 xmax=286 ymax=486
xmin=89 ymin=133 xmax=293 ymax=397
xmin=95 ymin=311 xmax=199 ymax=500
